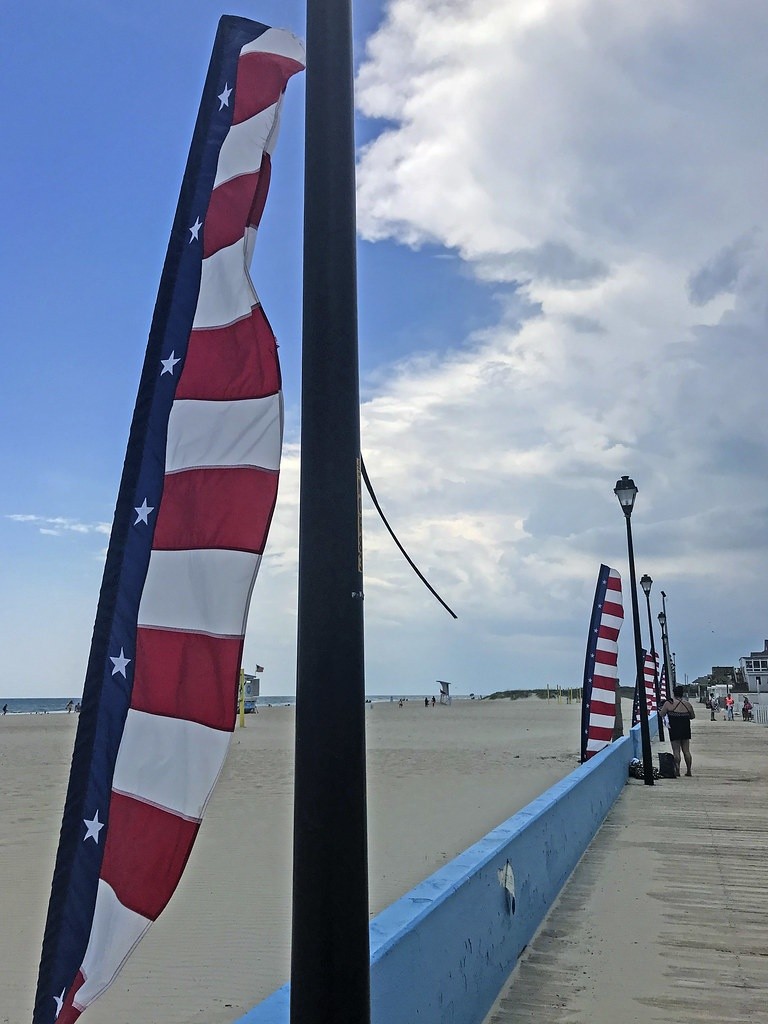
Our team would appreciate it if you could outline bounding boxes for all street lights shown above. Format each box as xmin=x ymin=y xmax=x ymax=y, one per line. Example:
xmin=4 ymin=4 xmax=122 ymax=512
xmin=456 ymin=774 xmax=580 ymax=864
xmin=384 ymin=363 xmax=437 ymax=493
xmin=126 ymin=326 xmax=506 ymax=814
xmin=614 ymin=474 xmax=656 ymax=787
xmin=657 ymin=611 xmax=672 ymax=700
xmin=640 ymin=573 xmax=666 ymax=743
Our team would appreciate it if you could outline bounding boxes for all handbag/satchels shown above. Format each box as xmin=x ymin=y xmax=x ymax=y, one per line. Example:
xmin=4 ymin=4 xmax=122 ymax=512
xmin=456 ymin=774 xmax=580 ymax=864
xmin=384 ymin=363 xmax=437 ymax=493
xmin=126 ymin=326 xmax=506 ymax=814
xmin=745 ymin=703 xmax=753 ymax=710
xmin=658 ymin=752 xmax=678 ymax=779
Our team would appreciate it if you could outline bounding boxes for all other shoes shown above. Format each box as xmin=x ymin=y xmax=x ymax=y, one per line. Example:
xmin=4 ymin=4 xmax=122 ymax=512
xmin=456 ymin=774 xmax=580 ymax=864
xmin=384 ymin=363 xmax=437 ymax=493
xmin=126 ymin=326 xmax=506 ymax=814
xmin=676 ymin=774 xmax=680 ymax=777
xmin=743 ymin=717 xmax=749 ymax=721
xmin=685 ymin=773 xmax=692 ymax=776
xmin=711 ymin=718 xmax=716 ymax=721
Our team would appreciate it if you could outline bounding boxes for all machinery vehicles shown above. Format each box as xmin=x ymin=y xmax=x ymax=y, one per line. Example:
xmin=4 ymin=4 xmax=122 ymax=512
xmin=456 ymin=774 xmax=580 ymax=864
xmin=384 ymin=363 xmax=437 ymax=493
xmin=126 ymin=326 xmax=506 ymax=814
xmin=237 ymin=671 xmax=261 ymax=715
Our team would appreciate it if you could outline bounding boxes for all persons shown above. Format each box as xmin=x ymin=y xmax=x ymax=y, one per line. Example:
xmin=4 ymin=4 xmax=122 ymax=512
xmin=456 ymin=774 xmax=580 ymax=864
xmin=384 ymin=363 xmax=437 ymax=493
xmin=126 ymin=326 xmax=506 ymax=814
xmin=425 ymin=696 xmax=436 ymax=707
xmin=2 ymin=704 xmax=8 ymax=715
xmin=659 ymin=685 xmax=696 ymax=776
xmin=66 ymin=700 xmax=81 ymax=713
xmin=710 ymin=693 xmax=717 ymax=721
xmin=725 ymin=694 xmax=734 ymax=721
xmin=743 ymin=701 xmax=750 ymax=721
xmin=398 ymin=699 xmax=402 ymax=708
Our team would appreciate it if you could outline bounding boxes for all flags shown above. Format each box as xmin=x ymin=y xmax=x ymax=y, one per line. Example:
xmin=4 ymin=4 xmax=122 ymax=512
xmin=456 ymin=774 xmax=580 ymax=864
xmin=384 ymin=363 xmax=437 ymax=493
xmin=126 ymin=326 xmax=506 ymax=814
xmin=255 ymin=664 xmax=263 ymax=672
